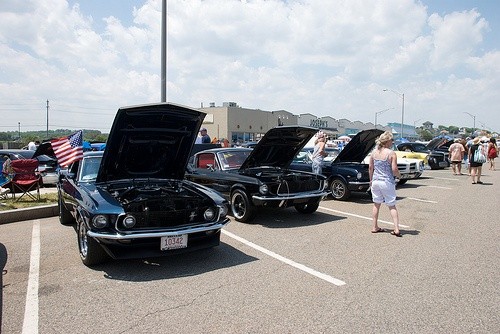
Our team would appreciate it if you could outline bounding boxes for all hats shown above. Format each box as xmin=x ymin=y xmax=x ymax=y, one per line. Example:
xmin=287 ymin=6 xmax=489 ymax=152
xmin=453 ymin=139 xmax=459 ymax=143
xmin=472 ymin=137 xmax=481 ymax=144
xmin=219 ymin=137 xmax=224 ymax=141
xmin=465 ymin=136 xmax=471 ymax=139
xmin=198 ymin=128 xmax=207 ymax=132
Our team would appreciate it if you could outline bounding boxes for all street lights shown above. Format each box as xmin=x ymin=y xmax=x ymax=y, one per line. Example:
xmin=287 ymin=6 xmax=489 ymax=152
xmin=383 ymin=89 xmax=404 ymax=138
xmin=464 ymin=111 xmax=476 ymax=128
xmin=375 ymin=108 xmax=396 ymax=129
xmin=17 ymin=122 xmax=20 ymax=139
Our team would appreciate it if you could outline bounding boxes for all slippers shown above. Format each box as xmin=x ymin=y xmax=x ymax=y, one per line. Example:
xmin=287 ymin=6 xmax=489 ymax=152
xmin=371 ymin=227 xmax=388 ymax=233
xmin=391 ymin=230 xmax=402 ymax=237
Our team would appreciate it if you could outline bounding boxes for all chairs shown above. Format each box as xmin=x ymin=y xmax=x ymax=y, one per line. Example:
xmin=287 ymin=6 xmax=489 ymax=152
xmin=8 ymin=159 xmax=40 ymax=204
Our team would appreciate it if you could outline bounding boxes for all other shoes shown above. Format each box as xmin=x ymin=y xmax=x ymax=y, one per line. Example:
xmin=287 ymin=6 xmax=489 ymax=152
xmin=472 ymin=181 xmax=475 ymax=184
xmin=477 ymin=181 xmax=482 ymax=184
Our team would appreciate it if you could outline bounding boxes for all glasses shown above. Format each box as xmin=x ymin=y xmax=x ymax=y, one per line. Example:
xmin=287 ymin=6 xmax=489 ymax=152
xmin=320 ymin=135 xmax=324 ymax=138
xmin=391 ymin=139 xmax=394 ymax=141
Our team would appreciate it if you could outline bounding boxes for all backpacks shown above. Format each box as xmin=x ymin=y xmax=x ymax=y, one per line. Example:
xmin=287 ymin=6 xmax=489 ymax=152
xmin=488 ymin=142 xmax=497 ymax=158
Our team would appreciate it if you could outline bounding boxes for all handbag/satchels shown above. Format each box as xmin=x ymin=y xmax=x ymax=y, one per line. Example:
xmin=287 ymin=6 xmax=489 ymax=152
xmin=474 ymin=145 xmax=486 ymax=163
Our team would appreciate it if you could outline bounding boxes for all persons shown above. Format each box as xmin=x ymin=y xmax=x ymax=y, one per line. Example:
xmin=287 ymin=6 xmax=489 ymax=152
xmin=28 ymin=140 xmax=40 ymax=151
xmin=487 ymin=138 xmax=498 ymax=171
xmin=312 ymin=133 xmax=328 ymax=174
xmin=448 ymin=139 xmax=465 ymax=175
xmin=224 ymin=138 xmax=229 ymax=147
xmin=369 ymin=130 xmax=403 ymax=238
xmin=200 ymin=128 xmax=210 ymax=144
xmin=468 ymin=137 xmax=483 ymax=184
xmin=443 ymin=139 xmax=449 ymax=147
xmin=461 ymin=135 xmax=477 ymax=176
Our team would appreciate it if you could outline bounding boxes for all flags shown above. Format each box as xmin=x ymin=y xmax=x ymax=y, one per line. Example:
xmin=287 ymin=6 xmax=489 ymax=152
xmin=51 ymin=130 xmax=84 ymax=167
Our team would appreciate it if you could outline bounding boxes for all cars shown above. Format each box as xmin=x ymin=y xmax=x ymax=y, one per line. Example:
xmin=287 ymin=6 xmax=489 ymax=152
xmin=239 ymin=133 xmax=499 ymax=202
xmin=0 ymin=100 xmax=232 ymax=268
xmin=182 ymin=124 xmax=333 ymax=223
